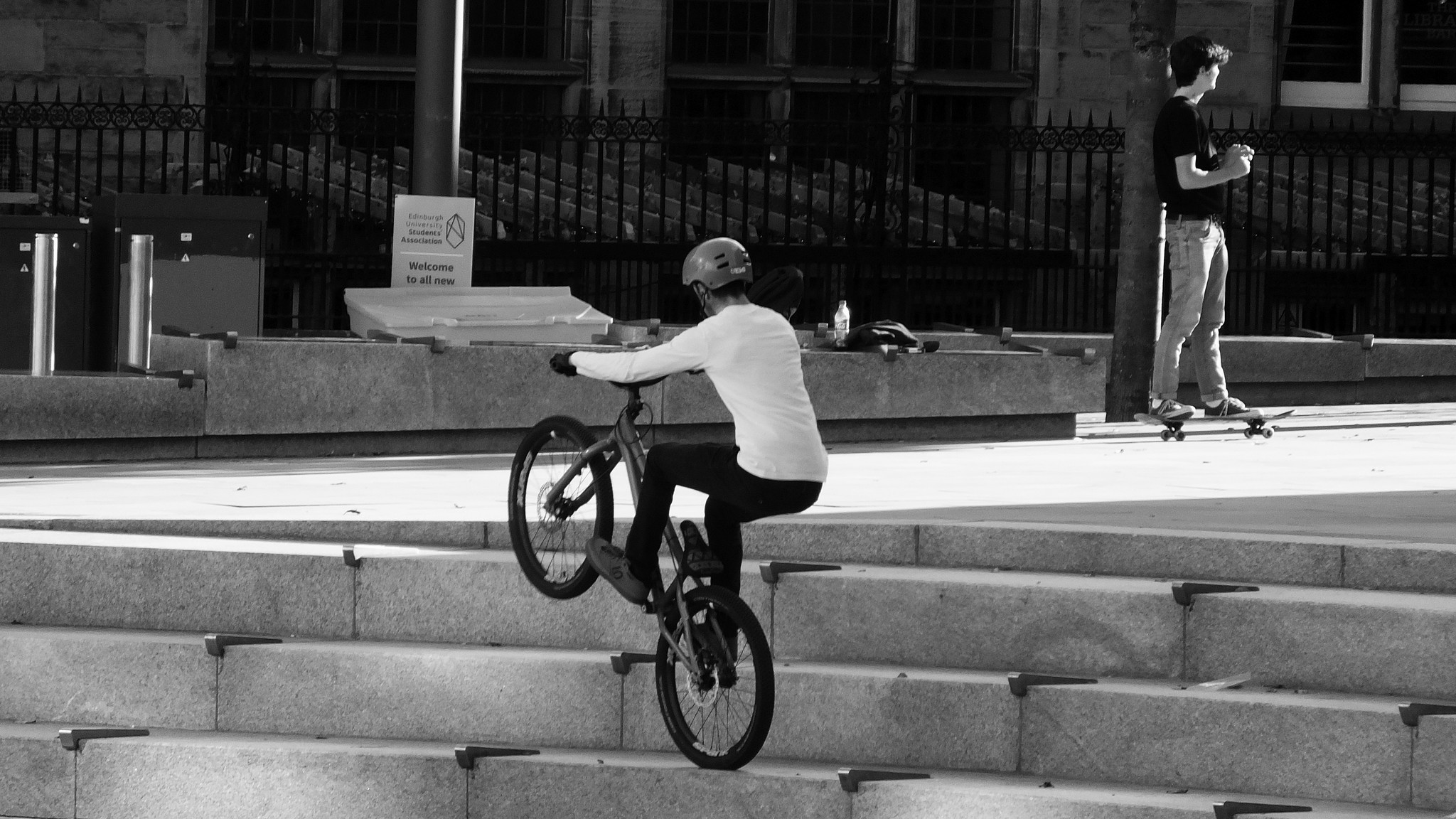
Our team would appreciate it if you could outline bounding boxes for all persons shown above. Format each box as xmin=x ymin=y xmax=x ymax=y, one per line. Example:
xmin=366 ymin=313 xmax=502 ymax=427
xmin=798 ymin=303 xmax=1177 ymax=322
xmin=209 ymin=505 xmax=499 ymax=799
xmin=549 ymin=236 xmax=829 ymax=688
xmin=1147 ymin=35 xmax=1264 ymax=419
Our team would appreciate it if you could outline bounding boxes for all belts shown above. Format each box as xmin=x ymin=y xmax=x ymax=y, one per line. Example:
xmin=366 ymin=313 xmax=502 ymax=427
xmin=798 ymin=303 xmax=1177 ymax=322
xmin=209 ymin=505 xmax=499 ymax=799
xmin=1167 ymin=211 xmax=1220 ymax=221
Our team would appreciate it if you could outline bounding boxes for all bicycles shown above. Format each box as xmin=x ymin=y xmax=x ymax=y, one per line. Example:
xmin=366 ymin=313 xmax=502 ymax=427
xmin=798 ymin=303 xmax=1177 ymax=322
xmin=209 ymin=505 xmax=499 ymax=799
xmin=507 ymin=345 xmax=780 ymax=773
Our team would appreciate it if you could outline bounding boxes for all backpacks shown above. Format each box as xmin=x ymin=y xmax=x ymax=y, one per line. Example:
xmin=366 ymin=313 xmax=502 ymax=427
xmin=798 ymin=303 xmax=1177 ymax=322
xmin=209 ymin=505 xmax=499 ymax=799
xmin=817 ymin=318 xmax=940 ymax=362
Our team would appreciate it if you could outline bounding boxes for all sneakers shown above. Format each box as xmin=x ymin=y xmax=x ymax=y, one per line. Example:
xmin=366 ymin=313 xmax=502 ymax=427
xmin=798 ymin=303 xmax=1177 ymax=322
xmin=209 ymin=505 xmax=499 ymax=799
xmin=1148 ymin=398 xmax=1196 ymax=422
xmin=1204 ymin=396 xmax=1265 ymax=419
xmin=586 ymin=535 xmax=651 ymax=605
xmin=692 ymin=621 xmax=738 ymax=661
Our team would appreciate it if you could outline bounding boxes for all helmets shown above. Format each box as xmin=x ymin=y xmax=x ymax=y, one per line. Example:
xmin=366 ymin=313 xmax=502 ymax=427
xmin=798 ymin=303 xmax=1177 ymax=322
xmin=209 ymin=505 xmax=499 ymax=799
xmin=682 ymin=237 xmax=754 ymax=292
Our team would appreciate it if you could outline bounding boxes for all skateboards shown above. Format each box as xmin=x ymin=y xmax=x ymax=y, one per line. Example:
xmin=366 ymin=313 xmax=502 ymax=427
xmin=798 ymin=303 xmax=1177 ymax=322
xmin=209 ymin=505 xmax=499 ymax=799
xmin=1134 ymin=409 xmax=1298 ymax=441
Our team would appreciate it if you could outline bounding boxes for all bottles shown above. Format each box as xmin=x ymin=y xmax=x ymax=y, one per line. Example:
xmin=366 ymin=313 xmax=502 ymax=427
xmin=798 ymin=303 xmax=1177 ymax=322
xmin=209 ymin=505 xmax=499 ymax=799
xmin=834 ymin=300 xmax=850 ymax=346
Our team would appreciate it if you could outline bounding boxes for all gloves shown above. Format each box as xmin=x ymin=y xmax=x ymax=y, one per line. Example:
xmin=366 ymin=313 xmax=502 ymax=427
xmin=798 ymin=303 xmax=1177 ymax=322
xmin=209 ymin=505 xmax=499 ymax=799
xmin=549 ymin=351 xmax=578 ymax=376
